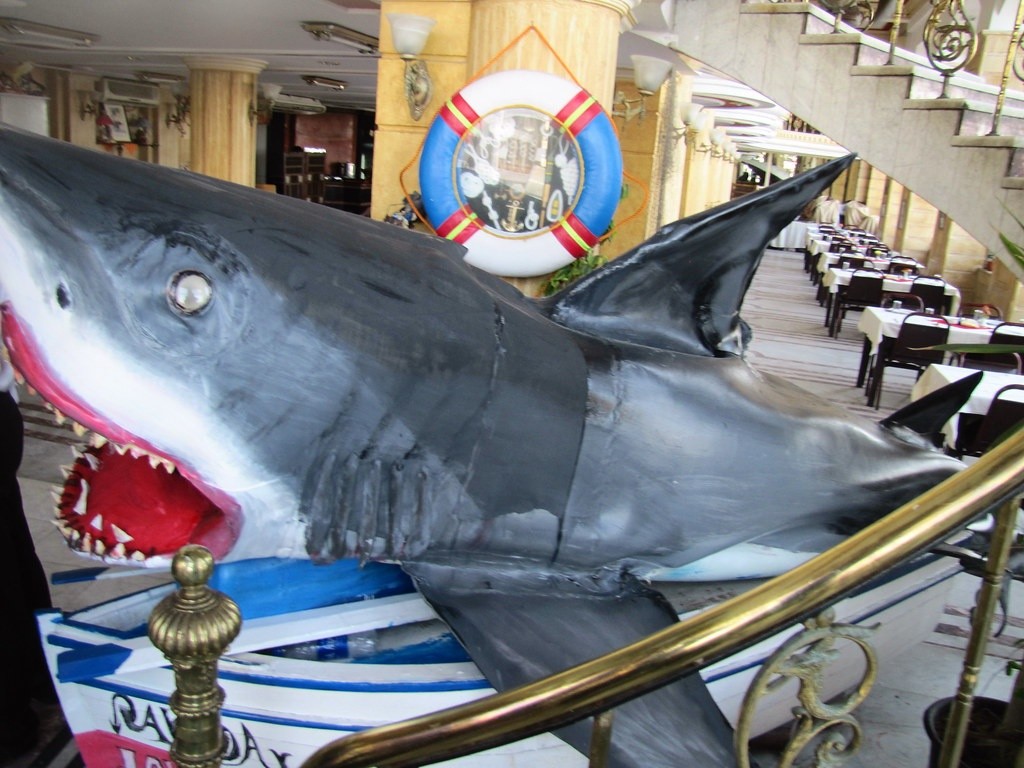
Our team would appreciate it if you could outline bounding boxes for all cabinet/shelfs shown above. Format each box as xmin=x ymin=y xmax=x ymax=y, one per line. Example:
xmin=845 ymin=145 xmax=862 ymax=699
xmin=282 ymin=151 xmax=326 ymax=204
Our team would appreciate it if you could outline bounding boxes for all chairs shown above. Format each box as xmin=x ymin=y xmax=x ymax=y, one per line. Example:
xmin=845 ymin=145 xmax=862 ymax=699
xmin=819 ymin=220 xmax=1024 ymax=464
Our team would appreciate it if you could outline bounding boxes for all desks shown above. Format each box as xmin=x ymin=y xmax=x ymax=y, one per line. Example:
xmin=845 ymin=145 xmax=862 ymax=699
xmin=769 ymin=220 xmax=841 ymax=252
xmin=821 ymin=268 xmax=962 ymax=337
xmin=812 ymin=239 xmax=901 ymax=281
xmin=911 ymin=363 xmax=1024 ymax=450
xmin=806 ymin=233 xmax=878 ymax=271
xmin=816 ymin=252 xmax=926 ymax=307
xmin=804 ymin=227 xmax=874 ymax=258
xmin=856 ymin=306 xmax=1024 ymax=407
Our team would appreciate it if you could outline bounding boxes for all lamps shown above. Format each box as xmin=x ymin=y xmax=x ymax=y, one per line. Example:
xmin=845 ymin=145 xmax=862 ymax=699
xmin=611 ymin=55 xmax=675 ymax=125
xmin=385 ymin=12 xmax=437 ymax=120
xmin=0 ymin=17 xmax=101 ymax=48
xmin=166 ymin=82 xmax=191 ymax=125
xmin=672 ymin=103 xmax=741 ymax=164
xmin=249 ymin=82 xmax=283 ymax=117
xmin=300 ymin=21 xmax=379 ymax=54
xmin=302 ymin=77 xmax=350 ymax=91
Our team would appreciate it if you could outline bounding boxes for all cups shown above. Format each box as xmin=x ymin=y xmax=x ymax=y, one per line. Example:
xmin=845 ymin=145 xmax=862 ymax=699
xmin=974 ymin=310 xmax=983 ymax=323
xmin=842 ymin=262 xmax=850 ymax=271
xmin=924 ymin=308 xmax=934 ymax=314
xmin=839 ymin=248 xmax=845 ymax=255
xmin=893 ymin=301 xmax=902 ymax=312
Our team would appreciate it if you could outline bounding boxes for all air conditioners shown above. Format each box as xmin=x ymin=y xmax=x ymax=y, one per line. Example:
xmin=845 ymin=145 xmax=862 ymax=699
xmin=95 ymin=78 xmax=160 ymax=108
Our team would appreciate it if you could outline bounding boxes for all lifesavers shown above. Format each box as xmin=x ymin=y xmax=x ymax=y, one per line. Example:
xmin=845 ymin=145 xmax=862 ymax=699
xmin=420 ymin=69 xmax=625 ymax=277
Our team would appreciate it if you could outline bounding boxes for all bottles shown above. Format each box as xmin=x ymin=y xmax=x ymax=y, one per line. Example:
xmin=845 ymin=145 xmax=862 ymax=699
xmin=273 ymin=630 xmax=375 ymax=661
